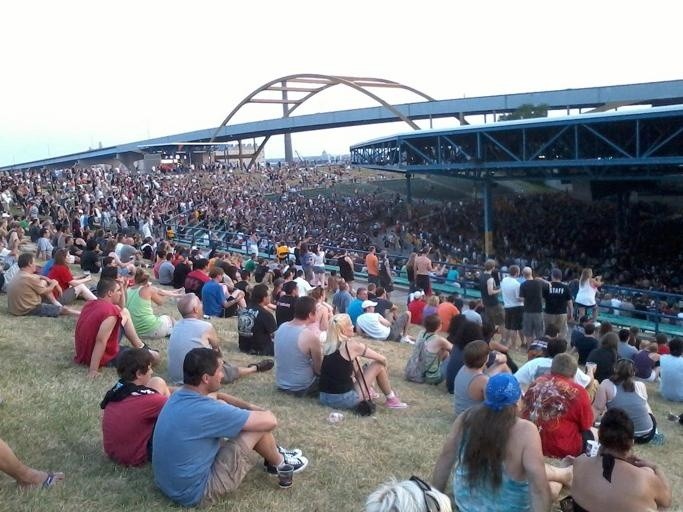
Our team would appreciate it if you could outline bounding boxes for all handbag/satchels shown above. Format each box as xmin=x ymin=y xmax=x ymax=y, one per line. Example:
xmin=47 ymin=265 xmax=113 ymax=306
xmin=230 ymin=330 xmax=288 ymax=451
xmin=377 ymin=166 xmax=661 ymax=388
xmin=424 ymin=368 xmax=444 ymax=385
xmin=354 ymin=400 xmax=378 ymax=416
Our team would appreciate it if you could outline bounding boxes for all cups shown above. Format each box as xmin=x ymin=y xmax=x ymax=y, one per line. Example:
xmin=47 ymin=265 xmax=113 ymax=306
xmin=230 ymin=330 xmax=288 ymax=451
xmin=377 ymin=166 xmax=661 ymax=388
xmin=277 ymin=464 xmax=294 ymax=488
xmin=584 ymin=361 xmax=598 ymax=374
xmin=329 ymin=413 xmax=343 ymax=423
xmin=83 ymin=270 xmax=90 ymax=275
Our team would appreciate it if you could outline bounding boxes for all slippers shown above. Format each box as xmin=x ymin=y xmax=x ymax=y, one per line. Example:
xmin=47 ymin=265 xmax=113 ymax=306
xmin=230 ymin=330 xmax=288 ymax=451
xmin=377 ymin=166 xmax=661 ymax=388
xmin=16 ymin=470 xmax=66 ymax=490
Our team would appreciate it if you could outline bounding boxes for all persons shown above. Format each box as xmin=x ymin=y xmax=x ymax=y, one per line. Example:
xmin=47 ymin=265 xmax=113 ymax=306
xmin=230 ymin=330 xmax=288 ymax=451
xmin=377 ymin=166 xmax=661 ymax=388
xmin=0 ymin=153 xmax=681 ymax=511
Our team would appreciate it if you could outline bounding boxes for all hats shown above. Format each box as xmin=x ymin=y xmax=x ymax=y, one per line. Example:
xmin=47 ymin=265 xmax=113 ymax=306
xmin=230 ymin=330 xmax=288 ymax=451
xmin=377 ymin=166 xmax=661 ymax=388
xmin=413 ymin=291 xmax=422 ymax=300
xmin=361 ymin=299 xmax=378 ymax=309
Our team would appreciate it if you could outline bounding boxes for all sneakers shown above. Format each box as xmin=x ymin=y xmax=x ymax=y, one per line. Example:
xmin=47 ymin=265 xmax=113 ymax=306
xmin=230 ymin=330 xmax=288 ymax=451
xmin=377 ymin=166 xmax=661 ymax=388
xmin=358 ymin=387 xmax=379 ymax=400
xmin=263 ymin=446 xmax=303 ymax=468
xmin=265 ymin=456 xmax=309 ymax=473
xmin=651 ymin=431 xmax=666 ymax=445
xmin=248 ymin=358 xmax=274 ymax=373
xmin=384 ymin=396 xmax=408 ymax=409
xmin=139 ymin=341 xmax=160 ymax=353
xmin=399 ymin=335 xmax=416 ymax=345
xmin=665 ymin=412 xmax=680 ymax=423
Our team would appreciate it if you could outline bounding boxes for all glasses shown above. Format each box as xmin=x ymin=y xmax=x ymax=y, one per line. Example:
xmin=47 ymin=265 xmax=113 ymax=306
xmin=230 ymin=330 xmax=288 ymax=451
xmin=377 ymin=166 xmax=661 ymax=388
xmin=407 ymin=474 xmax=441 ymax=512
